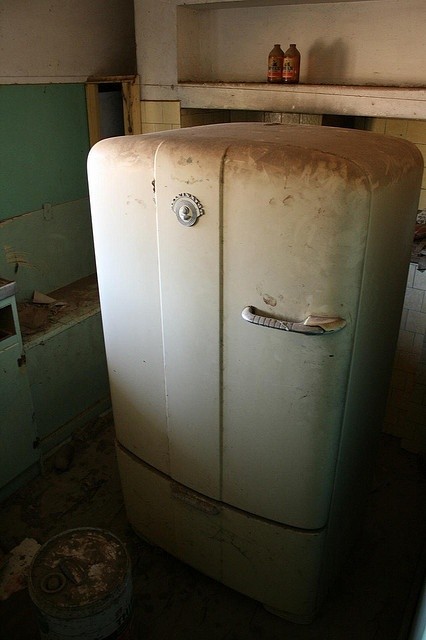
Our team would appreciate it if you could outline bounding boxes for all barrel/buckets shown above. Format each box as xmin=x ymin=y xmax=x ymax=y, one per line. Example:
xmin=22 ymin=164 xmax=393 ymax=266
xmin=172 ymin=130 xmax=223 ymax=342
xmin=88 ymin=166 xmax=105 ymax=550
xmin=27 ymin=526 xmax=135 ymax=638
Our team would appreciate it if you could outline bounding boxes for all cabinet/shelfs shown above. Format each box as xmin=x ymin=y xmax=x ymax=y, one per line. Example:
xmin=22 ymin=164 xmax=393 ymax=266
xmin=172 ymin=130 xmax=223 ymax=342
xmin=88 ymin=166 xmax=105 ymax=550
xmin=22 ymin=309 xmax=111 ymax=465
xmin=1 ymin=293 xmax=39 ymax=481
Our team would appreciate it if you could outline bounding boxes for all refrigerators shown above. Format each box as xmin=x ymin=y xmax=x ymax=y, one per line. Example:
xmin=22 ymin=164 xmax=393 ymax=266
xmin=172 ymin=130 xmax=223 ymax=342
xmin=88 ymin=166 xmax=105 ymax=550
xmin=85 ymin=120 xmax=425 ymax=626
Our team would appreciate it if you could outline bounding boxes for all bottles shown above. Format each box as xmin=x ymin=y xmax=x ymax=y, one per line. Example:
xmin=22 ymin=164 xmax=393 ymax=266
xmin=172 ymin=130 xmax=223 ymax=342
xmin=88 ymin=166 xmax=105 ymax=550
xmin=267 ymin=44 xmax=285 ymax=84
xmin=282 ymin=43 xmax=301 ymax=84
